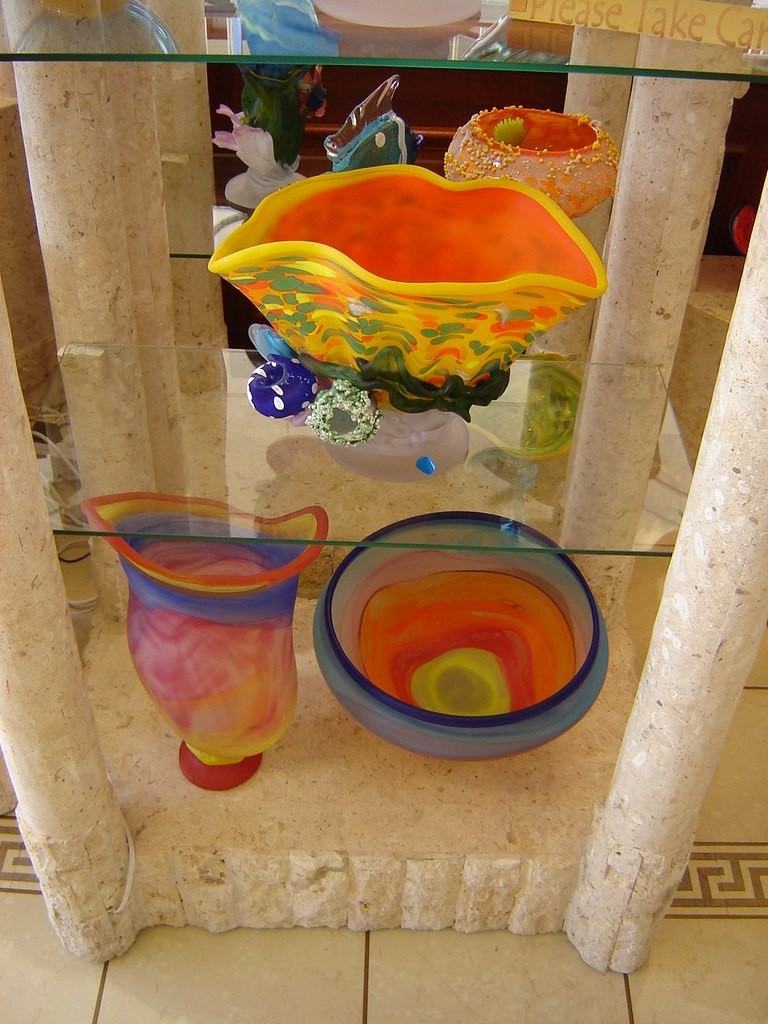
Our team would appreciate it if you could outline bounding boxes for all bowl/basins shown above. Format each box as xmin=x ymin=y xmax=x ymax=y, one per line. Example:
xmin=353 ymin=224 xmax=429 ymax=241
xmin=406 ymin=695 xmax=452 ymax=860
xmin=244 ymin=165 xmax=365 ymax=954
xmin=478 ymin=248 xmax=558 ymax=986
xmin=314 ymin=510 xmax=609 ymax=760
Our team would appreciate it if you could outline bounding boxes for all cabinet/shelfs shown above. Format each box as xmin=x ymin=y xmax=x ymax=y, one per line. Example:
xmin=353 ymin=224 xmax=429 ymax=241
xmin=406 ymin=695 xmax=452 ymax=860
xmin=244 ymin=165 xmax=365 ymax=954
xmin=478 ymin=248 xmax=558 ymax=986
xmin=0 ymin=0 xmax=766 ymax=975
